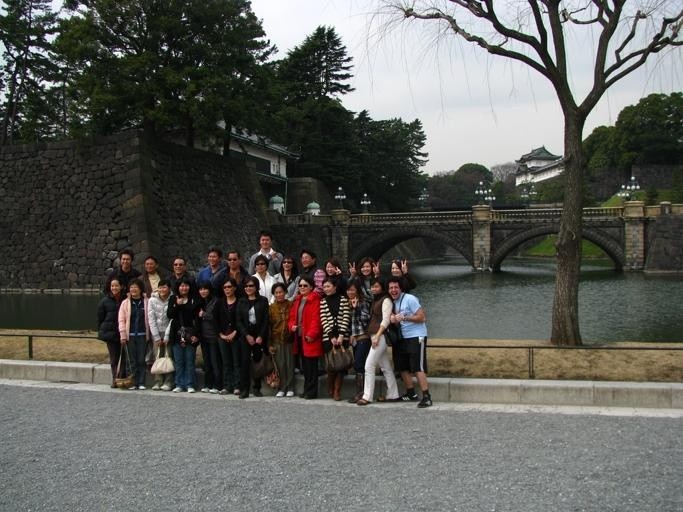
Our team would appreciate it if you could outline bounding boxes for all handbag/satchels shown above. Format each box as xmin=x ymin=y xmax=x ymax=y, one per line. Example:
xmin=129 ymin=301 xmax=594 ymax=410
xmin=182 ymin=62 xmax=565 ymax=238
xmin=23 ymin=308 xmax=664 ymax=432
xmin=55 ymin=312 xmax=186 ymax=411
xmin=324 ymin=343 xmax=353 ymax=374
xmin=150 ymin=343 xmax=176 ymax=375
xmin=176 ymin=325 xmax=201 ymax=348
xmin=114 ymin=373 xmax=135 ymax=389
xmin=242 ymin=344 xmax=274 ymax=379
xmin=263 ymin=354 xmax=281 ymax=389
xmin=201 ymin=298 xmax=224 ymax=341
xmin=383 ymin=322 xmax=402 ymax=346
xmin=97 ymin=322 xmax=121 ymax=344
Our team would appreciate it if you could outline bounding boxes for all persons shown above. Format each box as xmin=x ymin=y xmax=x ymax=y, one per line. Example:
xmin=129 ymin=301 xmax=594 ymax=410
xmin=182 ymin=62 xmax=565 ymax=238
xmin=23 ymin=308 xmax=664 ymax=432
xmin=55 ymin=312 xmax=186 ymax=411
xmin=93 ymin=227 xmax=434 ymax=410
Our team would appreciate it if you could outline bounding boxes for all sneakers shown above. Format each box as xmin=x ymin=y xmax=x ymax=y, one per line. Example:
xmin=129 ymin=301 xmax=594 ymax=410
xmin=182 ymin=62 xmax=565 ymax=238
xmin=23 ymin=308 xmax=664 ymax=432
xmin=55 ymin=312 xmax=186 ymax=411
xmin=110 ymin=382 xmax=117 ymax=388
xmin=286 ymin=390 xmax=294 ymax=397
xmin=200 ymin=388 xmax=264 ymax=398
xmin=298 ymin=393 xmax=317 ymax=399
xmin=128 ymin=385 xmax=137 ymax=390
xmin=152 ymin=381 xmax=160 ymax=390
xmin=356 ymin=398 xmax=368 ymax=405
xmin=187 ymin=387 xmax=196 ymax=393
xmin=138 ymin=385 xmax=146 ymax=390
xmin=160 ymin=383 xmax=172 ymax=390
xmin=377 ymin=392 xmax=433 ymax=408
xmin=172 ymin=386 xmax=185 ymax=393
xmin=275 ymin=390 xmax=285 ymax=397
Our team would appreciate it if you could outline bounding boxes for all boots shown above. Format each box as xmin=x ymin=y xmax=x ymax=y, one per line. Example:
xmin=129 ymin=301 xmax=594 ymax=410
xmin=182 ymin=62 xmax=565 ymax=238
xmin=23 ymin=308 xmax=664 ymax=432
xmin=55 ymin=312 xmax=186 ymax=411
xmin=333 ymin=374 xmax=343 ymax=400
xmin=348 ymin=373 xmax=364 ymax=403
xmin=328 ymin=372 xmax=334 ymax=398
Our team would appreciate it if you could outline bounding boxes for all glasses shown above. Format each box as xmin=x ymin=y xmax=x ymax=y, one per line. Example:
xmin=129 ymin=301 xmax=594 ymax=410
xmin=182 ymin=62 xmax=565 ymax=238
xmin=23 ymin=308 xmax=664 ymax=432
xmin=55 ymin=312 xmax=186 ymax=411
xmin=227 ymin=259 xmax=238 ymax=261
xmin=299 ymin=285 xmax=307 ymax=287
xmin=245 ymin=285 xmax=254 ymax=287
xmin=174 ymin=263 xmax=184 ymax=266
xmin=282 ymin=261 xmax=292 ymax=264
xmin=223 ymin=286 xmax=233 ymax=289
xmin=255 ymin=263 xmax=266 ymax=265
